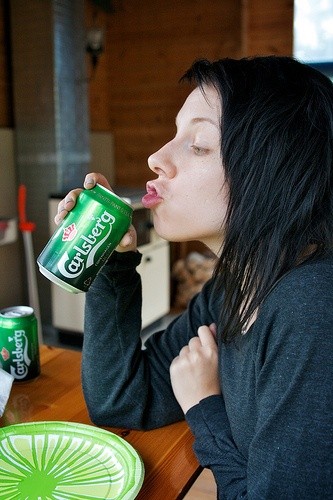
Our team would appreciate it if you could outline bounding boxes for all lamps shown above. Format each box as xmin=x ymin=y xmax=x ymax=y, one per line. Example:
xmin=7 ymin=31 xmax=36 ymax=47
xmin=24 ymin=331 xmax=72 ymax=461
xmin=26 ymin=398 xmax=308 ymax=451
xmin=72 ymin=24 xmax=104 ymax=83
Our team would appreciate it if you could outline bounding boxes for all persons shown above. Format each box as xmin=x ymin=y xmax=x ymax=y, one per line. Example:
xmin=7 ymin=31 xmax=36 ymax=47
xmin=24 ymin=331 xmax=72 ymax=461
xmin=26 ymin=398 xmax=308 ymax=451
xmin=52 ymin=53 xmax=331 ymax=499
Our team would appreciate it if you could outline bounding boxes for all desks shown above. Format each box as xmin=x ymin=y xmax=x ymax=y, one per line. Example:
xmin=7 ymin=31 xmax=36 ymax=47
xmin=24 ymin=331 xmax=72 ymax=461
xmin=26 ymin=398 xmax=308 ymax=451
xmin=0 ymin=344 xmax=204 ymax=500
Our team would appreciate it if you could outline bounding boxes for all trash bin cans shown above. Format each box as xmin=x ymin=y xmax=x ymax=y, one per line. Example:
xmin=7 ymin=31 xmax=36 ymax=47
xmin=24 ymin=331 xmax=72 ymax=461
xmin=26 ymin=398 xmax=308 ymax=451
xmin=44 ymin=189 xmax=170 ymax=350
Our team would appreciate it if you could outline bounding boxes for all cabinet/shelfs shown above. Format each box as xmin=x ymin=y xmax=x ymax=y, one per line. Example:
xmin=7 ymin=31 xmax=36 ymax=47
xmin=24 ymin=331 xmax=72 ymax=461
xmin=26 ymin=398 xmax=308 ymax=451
xmin=48 ymin=187 xmax=171 ymax=349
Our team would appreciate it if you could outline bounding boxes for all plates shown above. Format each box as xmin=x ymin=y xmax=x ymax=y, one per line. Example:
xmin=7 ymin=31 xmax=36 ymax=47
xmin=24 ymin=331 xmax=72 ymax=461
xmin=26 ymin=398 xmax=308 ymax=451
xmin=0 ymin=420 xmax=145 ymax=500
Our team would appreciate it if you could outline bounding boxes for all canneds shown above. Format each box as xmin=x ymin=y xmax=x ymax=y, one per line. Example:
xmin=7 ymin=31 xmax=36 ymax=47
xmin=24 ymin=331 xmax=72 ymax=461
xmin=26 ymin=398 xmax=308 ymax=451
xmin=0 ymin=306 xmax=41 ymax=383
xmin=37 ymin=183 xmax=134 ymax=294
